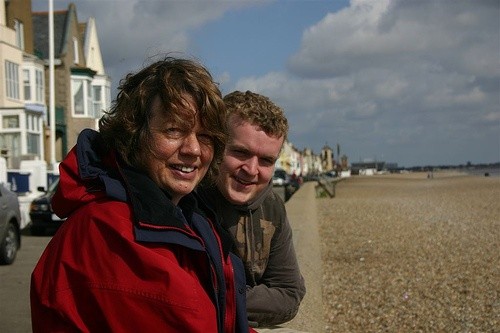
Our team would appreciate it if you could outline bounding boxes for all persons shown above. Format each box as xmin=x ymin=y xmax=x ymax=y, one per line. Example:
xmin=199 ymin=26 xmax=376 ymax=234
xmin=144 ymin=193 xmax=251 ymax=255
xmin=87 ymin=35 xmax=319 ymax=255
xmin=30 ymin=58 xmax=255 ymax=333
xmin=197 ymin=90 xmax=306 ymax=333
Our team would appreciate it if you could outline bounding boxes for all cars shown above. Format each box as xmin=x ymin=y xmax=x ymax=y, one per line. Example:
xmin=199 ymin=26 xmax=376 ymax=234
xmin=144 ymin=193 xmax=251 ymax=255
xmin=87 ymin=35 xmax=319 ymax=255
xmin=0 ymin=183 xmax=22 ymax=266
xmin=272 ymin=169 xmax=290 ymax=186
xmin=29 ymin=175 xmax=67 ymax=235
